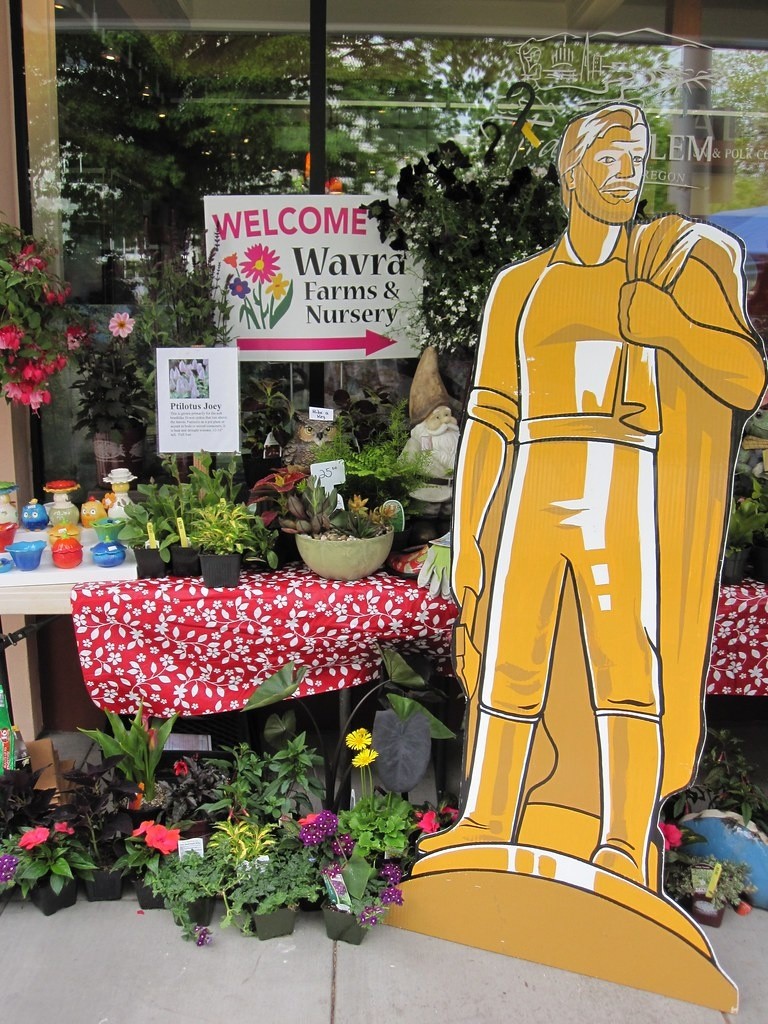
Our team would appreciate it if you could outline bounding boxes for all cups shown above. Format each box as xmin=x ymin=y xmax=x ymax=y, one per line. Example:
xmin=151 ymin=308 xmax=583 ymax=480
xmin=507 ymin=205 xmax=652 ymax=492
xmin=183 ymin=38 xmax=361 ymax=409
xmin=4 ymin=541 xmax=47 ymax=571
xmin=0 ymin=522 xmax=19 ymax=553
xmin=91 ymin=542 xmax=127 ymax=567
xmin=91 ymin=518 xmax=125 ymax=541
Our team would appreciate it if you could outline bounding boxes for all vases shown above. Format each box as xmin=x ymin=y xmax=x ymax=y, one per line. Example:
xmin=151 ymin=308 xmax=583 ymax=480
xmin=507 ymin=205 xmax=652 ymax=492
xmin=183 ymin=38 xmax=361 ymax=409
xmin=321 ymin=898 xmax=369 ymax=946
xmin=174 ymin=894 xmax=216 ymax=926
xmin=29 ymin=873 xmax=77 ymax=916
xmin=133 ymin=880 xmax=165 ymax=909
xmin=93 ymin=428 xmax=143 ymax=489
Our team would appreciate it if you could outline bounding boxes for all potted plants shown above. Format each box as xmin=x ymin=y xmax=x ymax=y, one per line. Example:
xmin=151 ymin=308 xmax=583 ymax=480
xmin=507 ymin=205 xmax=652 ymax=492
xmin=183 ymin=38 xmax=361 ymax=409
xmin=718 ymin=445 xmax=768 ymax=587
xmin=1 ymin=640 xmax=458 ymax=941
xmin=116 ymin=214 xmax=434 ymax=588
xmin=666 ymin=854 xmax=759 ymax=928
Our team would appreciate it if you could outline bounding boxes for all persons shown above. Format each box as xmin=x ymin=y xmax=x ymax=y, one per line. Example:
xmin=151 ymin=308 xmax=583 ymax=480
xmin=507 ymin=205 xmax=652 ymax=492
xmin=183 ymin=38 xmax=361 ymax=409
xmin=397 ymin=347 xmax=461 ymax=542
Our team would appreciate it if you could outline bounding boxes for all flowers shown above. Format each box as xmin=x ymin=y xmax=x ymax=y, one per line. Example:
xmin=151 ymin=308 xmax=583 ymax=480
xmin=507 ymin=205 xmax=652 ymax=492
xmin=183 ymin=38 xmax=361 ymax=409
xmin=0 ymin=821 xmax=100 ymax=900
xmin=335 ymin=727 xmax=413 ymax=858
xmin=153 ymin=849 xmax=233 ymax=946
xmin=108 ymin=817 xmax=197 ymax=887
xmin=71 ymin=306 xmax=150 ymax=439
xmin=659 ymin=820 xmax=709 ymax=897
xmin=0 ymin=210 xmax=98 ymax=419
xmin=297 ymin=809 xmax=405 ymax=928
xmin=408 ymin=799 xmax=459 ymax=858
